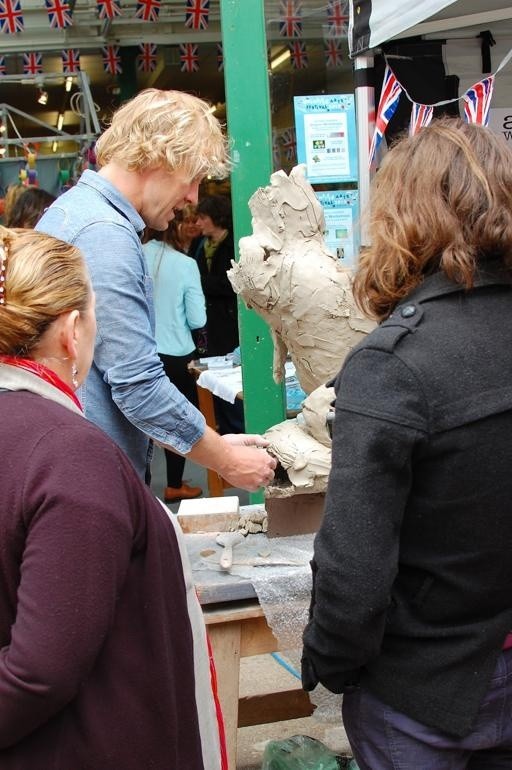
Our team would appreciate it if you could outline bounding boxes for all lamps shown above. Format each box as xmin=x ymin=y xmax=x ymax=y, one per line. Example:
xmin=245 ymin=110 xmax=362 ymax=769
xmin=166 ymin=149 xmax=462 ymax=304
xmin=33 ymin=82 xmax=50 ymax=104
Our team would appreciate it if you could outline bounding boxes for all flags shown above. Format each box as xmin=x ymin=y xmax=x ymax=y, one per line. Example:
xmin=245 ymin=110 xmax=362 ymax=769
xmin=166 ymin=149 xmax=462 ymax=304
xmin=0 ymin=0 xmax=224 ymax=74
xmin=280 ymin=127 xmax=299 ymax=165
xmin=279 ymin=0 xmax=349 ymax=68
xmin=359 ymin=65 xmax=403 ymax=175
xmin=460 ymin=75 xmax=497 ymax=130
xmin=270 ymin=128 xmax=283 ymax=167
xmin=407 ymin=99 xmax=437 ymax=139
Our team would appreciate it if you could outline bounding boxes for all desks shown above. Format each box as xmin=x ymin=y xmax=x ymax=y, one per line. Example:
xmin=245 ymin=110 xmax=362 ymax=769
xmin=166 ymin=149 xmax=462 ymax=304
xmin=176 ymin=494 xmax=320 ymax=770
xmin=176 ymin=353 xmax=310 ymax=502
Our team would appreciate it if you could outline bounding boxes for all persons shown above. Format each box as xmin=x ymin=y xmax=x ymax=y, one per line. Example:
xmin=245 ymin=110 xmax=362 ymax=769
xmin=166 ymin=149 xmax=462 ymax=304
xmin=4 ymin=185 xmax=59 ymax=231
xmin=187 ymin=194 xmax=246 ymax=437
xmin=296 ymin=116 xmax=510 ymax=770
xmin=0 ymin=223 xmax=233 ymax=769
xmin=33 ymin=84 xmax=281 ymax=493
xmin=174 ymin=201 xmax=204 ymax=253
xmin=137 ymin=221 xmax=210 ymax=505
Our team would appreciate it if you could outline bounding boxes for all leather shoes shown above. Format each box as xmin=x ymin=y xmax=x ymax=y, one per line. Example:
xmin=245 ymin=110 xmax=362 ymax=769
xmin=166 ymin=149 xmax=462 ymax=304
xmin=165 ymin=484 xmax=202 ymax=504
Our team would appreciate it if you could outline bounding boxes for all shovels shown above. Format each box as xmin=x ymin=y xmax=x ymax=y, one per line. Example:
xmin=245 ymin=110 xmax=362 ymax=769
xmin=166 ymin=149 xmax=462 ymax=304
xmin=217 ymin=532 xmax=244 ymax=568
